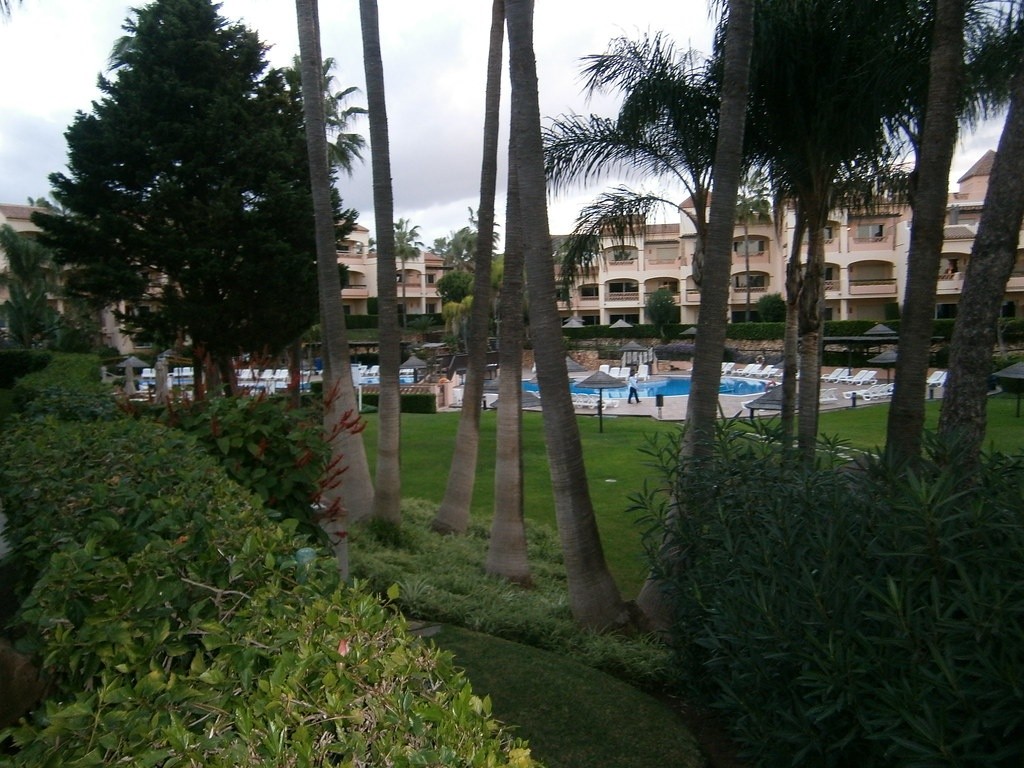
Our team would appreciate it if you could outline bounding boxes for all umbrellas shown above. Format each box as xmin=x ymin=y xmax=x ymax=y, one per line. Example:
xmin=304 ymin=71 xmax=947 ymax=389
xmin=399 ymin=356 xmax=428 ymax=384
xmin=866 ymin=349 xmax=898 ymax=384
xmin=528 ymin=372 xmax=576 ymax=388
xmin=490 ymin=391 xmax=545 ymax=411
xmin=115 ymin=355 xmax=150 ymax=377
xmin=565 ymin=352 xmax=590 ymax=372
xmin=746 ymin=383 xmax=800 ymax=411
xmin=618 ymin=341 xmax=647 ymax=369
xmin=991 ymin=361 xmax=1024 ymax=417
xmin=863 ymin=324 xmax=897 ymax=334
xmin=679 ymin=327 xmax=697 ymax=345
xmin=561 ymin=315 xmax=586 ymax=349
xmin=609 ymin=318 xmax=634 ymax=346
xmin=573 ymin=371 xmax=627 ymax=433
xmin=772 ymin=352 xmax=802 ymax=370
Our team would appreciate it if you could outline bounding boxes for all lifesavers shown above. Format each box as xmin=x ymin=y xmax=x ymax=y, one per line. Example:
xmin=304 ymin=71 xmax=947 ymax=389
xmin=756 ymin=355 xmax=765 ymax=365
xmin=766 ymin=381 xmax=776 ymax=394
xmin=439 ymin=377 xmax=449 ymax=392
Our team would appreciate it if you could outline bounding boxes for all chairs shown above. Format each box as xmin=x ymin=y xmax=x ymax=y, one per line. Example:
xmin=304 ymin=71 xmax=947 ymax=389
xmin=141 ymin=364 xmax=378 ymax=382
xmin=570 ymin=393 xmax=619 ymax=411
xmin=687 ymin=362 xmax=947 ymax=401
xmin=599 ymin=364 xmax=649 ymax=384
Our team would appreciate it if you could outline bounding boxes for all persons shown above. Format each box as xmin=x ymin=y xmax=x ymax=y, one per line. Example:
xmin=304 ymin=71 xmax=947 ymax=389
xmin=627 ymin=369 xmax=642 ymax=404
xmin=944 ymin=262 xmax=958 ymax=280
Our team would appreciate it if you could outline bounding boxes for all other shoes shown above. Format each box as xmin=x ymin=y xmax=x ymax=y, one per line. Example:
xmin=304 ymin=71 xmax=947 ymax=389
xmin=637 ymin=401 xmax=642 ymax=403
xmin=629 ymin=402 xmax=633 ymax=404
